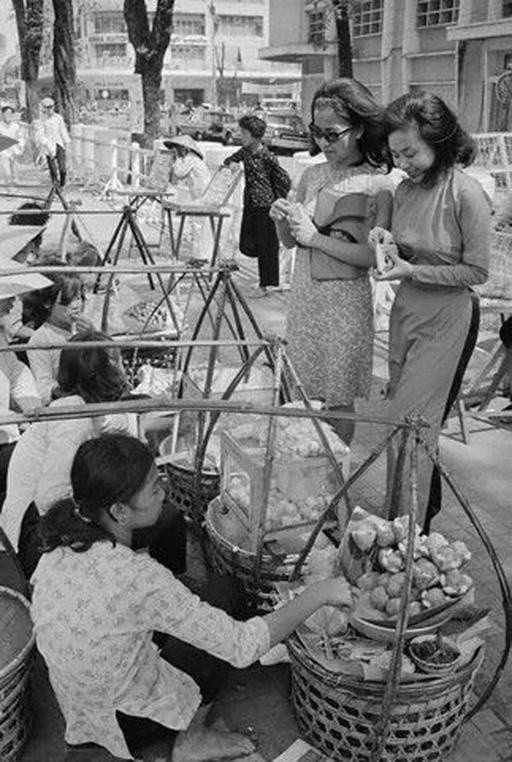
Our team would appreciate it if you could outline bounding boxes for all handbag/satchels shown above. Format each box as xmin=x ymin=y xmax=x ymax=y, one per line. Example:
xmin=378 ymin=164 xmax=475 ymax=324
xmin=240 ymin=207 xmax=273 ymax=257
xmin=308 ymin=187 xmax=368 ymax=279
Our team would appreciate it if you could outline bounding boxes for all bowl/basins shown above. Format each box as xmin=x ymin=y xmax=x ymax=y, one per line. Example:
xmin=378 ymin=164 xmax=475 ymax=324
xmin=407 ymin=635 xmax=465 ymax=673
xmin=349 ymin=613 xmax=451 ymax=645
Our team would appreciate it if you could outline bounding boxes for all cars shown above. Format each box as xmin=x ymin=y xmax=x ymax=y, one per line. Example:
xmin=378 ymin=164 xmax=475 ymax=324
xmin=172 ymin=107 xmax=237 ymax=143
xmin=220 ymin=107 xmax=312 ymax=155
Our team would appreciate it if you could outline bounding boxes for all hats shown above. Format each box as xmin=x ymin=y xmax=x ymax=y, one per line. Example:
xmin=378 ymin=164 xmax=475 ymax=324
xmin=163 ymin=135 xmax=206 ymax=161
xmin=1 ymin=224 xmax=53 ymax=298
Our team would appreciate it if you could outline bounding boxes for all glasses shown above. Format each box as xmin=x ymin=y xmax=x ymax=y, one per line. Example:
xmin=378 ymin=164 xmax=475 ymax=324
xmin=308 ymin=122 xmax=354 ymax=141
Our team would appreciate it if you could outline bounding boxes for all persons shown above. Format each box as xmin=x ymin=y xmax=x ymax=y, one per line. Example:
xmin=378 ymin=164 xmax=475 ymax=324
xmin=164 ymin=134 xmax=216 ymax=263
xmin=1 ymin=201 xmax=354 ymax=761
xmin=1 ymin=97 xmax=68 ymax=189
xmin=268 ymin=78 xmax=396 ymax=446
xmin=220 ymin=101 xmax=298 ymax=300
xmin=367 ymin=91 xmax=489 ymax=535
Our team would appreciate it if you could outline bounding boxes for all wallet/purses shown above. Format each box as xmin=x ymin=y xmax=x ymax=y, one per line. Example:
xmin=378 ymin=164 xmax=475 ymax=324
xmin=275 ymin=204 xmax=304 ymax=248
xmin=375 ymin=241 xmax=398 ymax=272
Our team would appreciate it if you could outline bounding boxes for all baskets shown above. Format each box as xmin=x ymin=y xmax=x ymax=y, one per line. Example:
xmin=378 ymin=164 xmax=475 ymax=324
xmin=0 ymin=526 xmax=39 ymax=762
xmin=194 ymin=337 xmax=353 ymax=622
xmin=284 ymin=413 xmax=512 ymax=762
xmin=160 ymin=269 xmax=294 ymax=520
xmin=158 ymin=421 xmax=511 ymax=762
xmin=100 ymin=312 xmax=182 ymax=392
